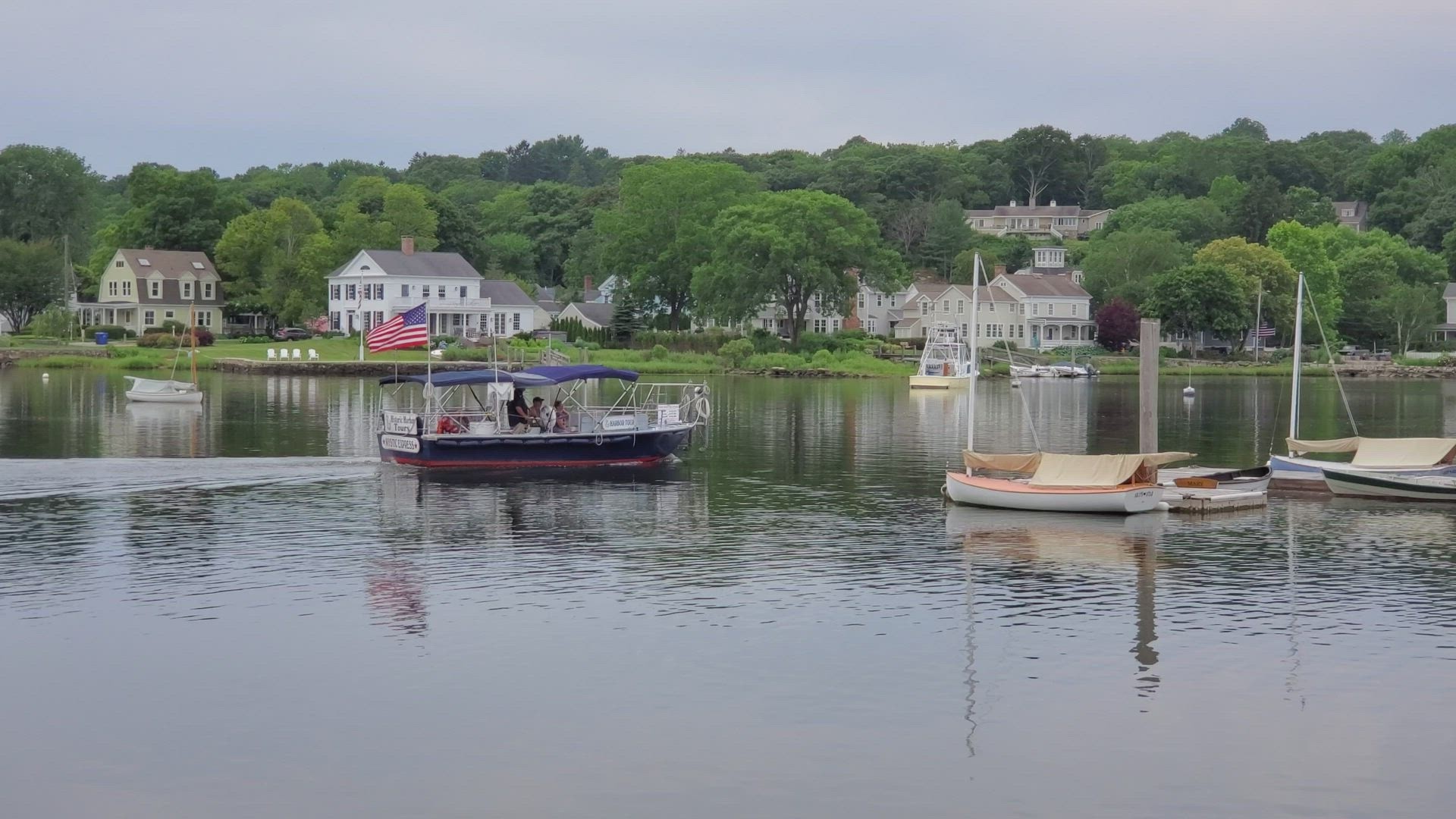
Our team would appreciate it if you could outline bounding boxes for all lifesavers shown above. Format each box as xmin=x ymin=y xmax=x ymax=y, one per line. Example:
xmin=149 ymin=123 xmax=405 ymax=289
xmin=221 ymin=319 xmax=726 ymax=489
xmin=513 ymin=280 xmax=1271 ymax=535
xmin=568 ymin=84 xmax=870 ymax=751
xmin=655 ymin=432 xmax=676 ymax=455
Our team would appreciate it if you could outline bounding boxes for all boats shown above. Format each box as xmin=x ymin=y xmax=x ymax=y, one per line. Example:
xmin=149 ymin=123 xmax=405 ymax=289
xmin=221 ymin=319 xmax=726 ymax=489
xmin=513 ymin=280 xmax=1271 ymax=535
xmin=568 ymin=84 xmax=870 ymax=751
xmin=1031 ymin=361 xmax=1100 ymax=377
xmin=1155 ymin=462 xmax=1273 ymax=510
xmin=1008 ymin=365 xmax=1040 ymax=377
xmin=371 ymin=333 xmax=712 ymax=471
xmin=1321 ymin=437 xmax=1456 ymax=500
xmin=907 ymin=320 xmax=981 ymax=390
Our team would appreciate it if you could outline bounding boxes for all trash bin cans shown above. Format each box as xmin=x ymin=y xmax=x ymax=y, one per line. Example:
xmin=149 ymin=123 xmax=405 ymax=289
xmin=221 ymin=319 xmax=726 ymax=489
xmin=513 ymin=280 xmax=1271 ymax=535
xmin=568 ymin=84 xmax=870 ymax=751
xmin=95 ymin=332 xmax=109 ymax=345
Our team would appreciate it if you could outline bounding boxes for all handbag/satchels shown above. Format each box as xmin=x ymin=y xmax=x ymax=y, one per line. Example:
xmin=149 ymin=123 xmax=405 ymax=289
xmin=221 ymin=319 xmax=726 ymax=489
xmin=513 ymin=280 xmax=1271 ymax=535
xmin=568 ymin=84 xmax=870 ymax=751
xmin=514 ymin=422 xmax=531 ymax=434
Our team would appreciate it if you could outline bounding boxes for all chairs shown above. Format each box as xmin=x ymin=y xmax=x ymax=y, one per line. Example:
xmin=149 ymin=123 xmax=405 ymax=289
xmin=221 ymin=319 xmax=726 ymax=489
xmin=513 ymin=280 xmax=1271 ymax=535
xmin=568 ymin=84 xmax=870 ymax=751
xmin=291 ymin=348 xmax=302 ymax=361
xmin=307 ymin=348 xmax=320 ymax=361
xmin=267 ymin=347 xmax=278 ymax=361
xmin=279 ymin=347 xmax=290 ymax=361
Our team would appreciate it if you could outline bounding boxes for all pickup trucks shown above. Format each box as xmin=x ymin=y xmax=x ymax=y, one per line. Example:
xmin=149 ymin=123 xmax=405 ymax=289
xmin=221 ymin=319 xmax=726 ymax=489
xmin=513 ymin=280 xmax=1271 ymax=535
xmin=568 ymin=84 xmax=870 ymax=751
xmin=1336 ymin=345 xmax=1371 ymax=356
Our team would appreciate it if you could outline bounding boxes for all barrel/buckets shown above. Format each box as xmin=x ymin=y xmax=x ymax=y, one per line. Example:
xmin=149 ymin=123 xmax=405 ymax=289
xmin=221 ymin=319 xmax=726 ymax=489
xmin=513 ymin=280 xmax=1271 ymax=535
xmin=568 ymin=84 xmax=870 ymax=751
xmin=469 ymin=422 xmax=496 ymax=435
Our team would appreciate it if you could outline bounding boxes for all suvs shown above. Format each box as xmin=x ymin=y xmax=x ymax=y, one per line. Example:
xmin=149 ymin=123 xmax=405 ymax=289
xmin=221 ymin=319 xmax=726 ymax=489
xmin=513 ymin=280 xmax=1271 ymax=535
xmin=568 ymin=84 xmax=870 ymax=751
xmin=275 ymin=327 xmax=313 ymax=343
xmin=1363 ymin=348 xmax=1392 ymax=360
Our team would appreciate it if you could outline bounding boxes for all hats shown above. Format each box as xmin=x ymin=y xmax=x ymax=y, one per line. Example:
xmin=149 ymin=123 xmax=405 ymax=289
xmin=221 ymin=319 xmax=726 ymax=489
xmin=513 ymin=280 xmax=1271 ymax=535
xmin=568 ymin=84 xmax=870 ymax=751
xmin=533 ymin=397 xmax=545 ymax=403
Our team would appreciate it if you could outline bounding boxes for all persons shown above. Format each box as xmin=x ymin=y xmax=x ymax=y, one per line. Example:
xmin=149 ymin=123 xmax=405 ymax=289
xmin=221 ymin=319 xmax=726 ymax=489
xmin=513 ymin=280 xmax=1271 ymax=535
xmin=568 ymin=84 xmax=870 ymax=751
xmin=505 ymin=388 xmax=530 ymax=435
xmin=552 ymin=401 xmax=570 ymax=432
xmin=528 ymin=397 xmax=550 ymax=433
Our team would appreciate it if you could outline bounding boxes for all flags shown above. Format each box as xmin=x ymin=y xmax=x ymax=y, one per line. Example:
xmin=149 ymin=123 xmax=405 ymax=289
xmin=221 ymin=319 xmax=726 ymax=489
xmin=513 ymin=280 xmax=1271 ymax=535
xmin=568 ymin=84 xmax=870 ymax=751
xmin=1251 ymin=318 xmax=1276 ymax=337
xmin=365 ymin=302 xmax=428 ymax=354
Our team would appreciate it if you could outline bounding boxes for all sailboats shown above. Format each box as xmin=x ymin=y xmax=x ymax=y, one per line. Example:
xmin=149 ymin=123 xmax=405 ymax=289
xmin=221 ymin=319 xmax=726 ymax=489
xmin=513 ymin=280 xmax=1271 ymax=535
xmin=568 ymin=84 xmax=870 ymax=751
xmin=1260 ymin=271 xmax=1382 ymax=489
xmin=945 ymin=255 xmax=1198 ymax=514
xmin=123 ymin=300 xmax=203 ymax=402
